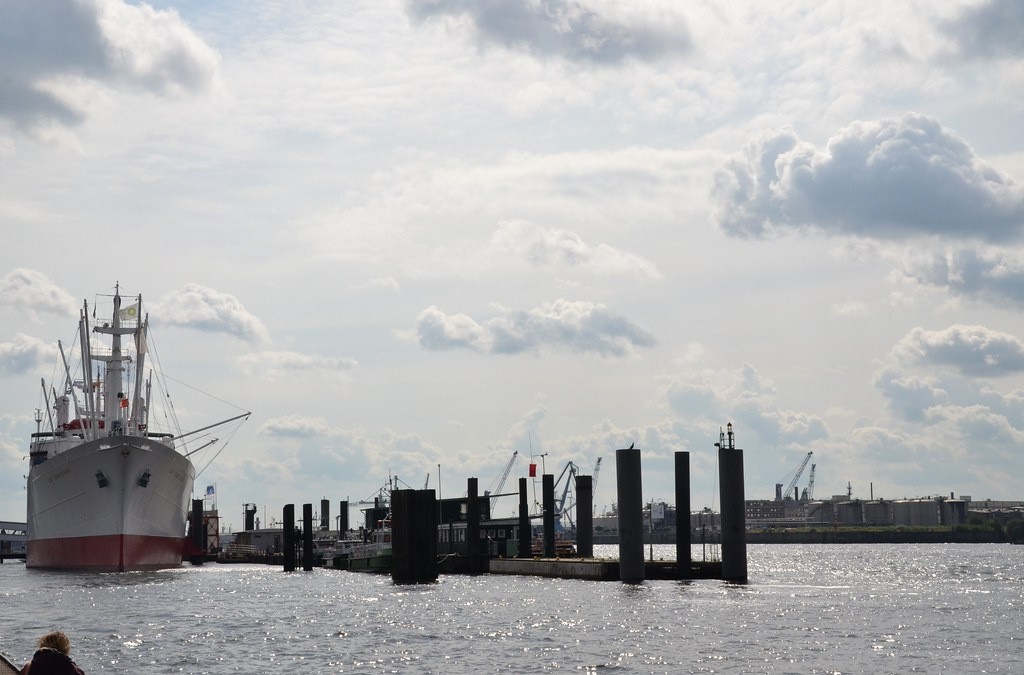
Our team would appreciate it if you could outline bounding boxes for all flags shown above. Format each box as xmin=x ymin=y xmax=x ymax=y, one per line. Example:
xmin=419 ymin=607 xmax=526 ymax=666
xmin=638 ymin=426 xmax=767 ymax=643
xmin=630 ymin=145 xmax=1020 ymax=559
xmin=529 ymin=464 xmax=536 ymax=477
xmin=120 ymin=300 xmax=138 ymax=321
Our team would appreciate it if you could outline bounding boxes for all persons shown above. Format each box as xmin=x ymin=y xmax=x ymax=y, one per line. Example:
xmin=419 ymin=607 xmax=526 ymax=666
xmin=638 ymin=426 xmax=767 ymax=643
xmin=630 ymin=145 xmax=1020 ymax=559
xmin=20 ymin=632 xmax=85 ymax=675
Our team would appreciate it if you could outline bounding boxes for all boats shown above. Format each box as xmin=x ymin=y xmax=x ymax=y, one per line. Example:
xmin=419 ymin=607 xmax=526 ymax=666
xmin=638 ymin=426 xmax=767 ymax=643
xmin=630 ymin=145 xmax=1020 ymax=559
xmin=252 ymin=475 xmax=393 ymax=576
xmin=25 ymin=279 xmax=254 ymax=573
xmin=533 ymin=532 xmax=577 ymax=558
